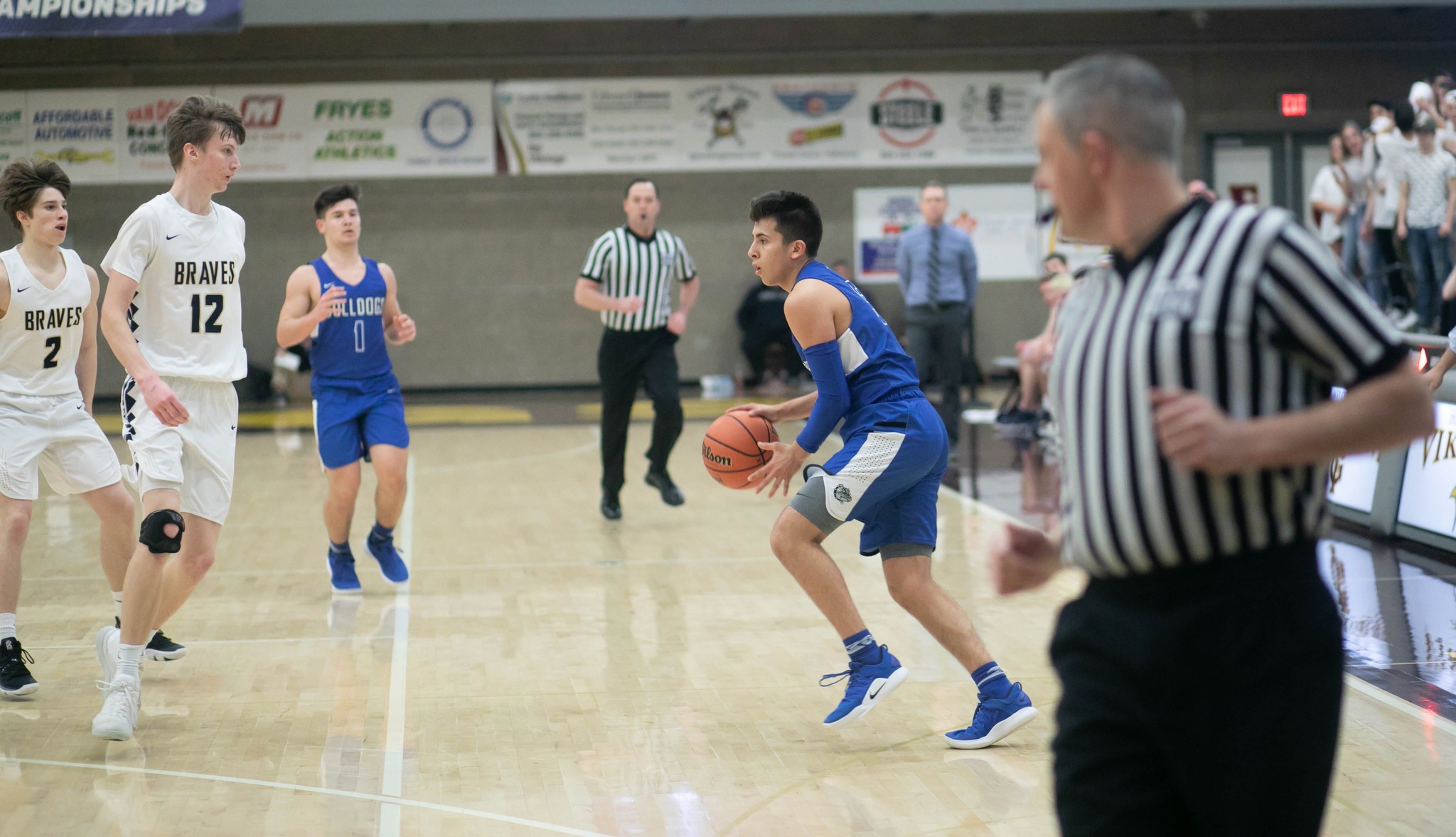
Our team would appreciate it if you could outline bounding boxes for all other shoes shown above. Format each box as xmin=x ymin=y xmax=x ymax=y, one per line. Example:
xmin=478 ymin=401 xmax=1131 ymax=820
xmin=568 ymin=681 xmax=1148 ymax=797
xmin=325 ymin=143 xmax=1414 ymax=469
xmin=602 ymin=490 xmax=621 ymax=519
xmin=645 ymin=466 xmax=684 ymax=505
xmin=998 ymin=407 xmax=1037 ymax=423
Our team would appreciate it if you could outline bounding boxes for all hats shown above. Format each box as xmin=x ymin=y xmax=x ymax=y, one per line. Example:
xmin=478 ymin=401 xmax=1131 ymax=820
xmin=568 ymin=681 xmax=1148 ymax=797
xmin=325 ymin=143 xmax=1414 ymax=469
xmin=1413 ymin=114 xmax=1436 ymax=128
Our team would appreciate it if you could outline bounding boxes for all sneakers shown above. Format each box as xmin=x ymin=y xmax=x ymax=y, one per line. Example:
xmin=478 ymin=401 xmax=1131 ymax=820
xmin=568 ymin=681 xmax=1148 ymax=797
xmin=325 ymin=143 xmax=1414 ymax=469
xmin=96 ymin=626 xmax=145 ymax=711
xmin=0 ymin=637 xmax=39 ymax=695
xmin=92 ymin=675 xmax=140 ymax=740
xmin=115 ymin=616 xmax=187 ymax=661
xmin=819 ymin=644 xmax=909 ymax=728
xmin=328 ymin=546 xmax=361 ymax=591
xmin=366 ymin=526 xmax=409 ymax=583
xmin=945 ymin=682 xmax=1038 ymax=749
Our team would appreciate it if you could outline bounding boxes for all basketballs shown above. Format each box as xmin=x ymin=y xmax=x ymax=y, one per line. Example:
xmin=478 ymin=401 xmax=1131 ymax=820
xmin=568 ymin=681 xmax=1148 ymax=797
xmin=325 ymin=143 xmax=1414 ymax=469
xmin=701 ymin=410 xmax=781 ymax=491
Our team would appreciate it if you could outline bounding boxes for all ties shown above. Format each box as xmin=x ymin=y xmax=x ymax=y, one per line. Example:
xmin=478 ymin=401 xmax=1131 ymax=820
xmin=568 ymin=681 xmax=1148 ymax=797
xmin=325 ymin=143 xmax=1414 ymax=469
xmin=927 ymin=228 xmax=939 ymax=306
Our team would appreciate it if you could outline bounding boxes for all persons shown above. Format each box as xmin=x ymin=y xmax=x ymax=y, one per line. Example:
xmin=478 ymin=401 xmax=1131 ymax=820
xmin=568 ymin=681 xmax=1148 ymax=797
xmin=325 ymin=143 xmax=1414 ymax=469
xmin=90 ymin=93 xmax=249 ymax=743
xmin=726 ymin=189 xmax=1038 ymax=750
xmin=574 ymin=178 xmax=702 ymax=520
xmin=890 ymin=179 xmax=982 ymax=446
xmin=986 ymin=50 xmax=1439 ymax=837
xmin=276 ymin=182 xmax=418 ymax=597
xmin=0 ymin=155 xmax=188 ymax=701
xmin=994 ymin=66 xmax=1456 ymax=435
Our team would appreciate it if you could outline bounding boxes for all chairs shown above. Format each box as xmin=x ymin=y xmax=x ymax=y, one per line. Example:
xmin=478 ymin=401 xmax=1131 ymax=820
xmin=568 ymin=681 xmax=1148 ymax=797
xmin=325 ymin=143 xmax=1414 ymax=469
xmin=963 ymin=306 xmax=1019 ymax=475
